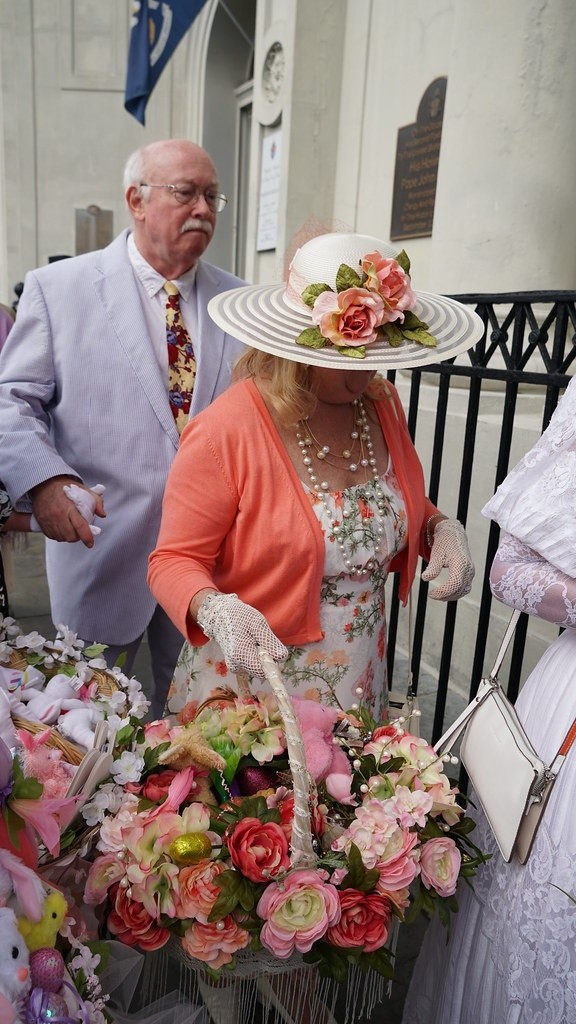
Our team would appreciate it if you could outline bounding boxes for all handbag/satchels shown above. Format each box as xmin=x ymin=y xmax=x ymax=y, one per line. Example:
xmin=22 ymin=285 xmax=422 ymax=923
xmin=433 ymin=676 xmax=557 ymax=865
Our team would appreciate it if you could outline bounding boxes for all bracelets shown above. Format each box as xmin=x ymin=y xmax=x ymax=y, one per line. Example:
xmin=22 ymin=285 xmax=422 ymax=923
xmin=427 ymin=514 xmax=440 ymax=548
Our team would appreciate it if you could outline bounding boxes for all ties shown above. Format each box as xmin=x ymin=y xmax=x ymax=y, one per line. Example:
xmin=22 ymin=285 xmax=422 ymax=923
xmin=162 ymin=281 xmax=198 ymax=436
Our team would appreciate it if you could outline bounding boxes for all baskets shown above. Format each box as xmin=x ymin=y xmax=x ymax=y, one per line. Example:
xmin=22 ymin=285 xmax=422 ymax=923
xmin=0 ymin=629 xmax=482 ymax=979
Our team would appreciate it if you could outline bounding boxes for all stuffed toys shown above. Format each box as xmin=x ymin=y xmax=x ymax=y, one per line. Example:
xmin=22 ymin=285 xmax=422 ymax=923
xmin=18 ymin=675 xmax=106 ymax=798
xmin=158 ymin=731 xmax=228 ymax=807
xmin=0 ymin=847 xmax=68 ymax=1024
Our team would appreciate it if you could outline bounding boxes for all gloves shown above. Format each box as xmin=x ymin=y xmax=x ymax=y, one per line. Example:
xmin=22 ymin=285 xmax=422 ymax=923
xmin=30 ymin=484 xmax=105 ymax=536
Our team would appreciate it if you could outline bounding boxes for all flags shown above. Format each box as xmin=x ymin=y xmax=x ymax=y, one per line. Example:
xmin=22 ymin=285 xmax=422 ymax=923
xmin=124 ymin=0 xmax=207 ymax=127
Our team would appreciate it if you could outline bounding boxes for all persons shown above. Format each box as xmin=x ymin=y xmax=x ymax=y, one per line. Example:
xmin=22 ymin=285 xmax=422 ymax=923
xmin=0 ymin=139 xmax=256 ymax=724
xmin=398 ymin=372 xmax=576 ymax=1024
xmin=144 ymin=234 xmax=486 ymax=1024
xmin=0 ymin=479 xmax=106 ymax=747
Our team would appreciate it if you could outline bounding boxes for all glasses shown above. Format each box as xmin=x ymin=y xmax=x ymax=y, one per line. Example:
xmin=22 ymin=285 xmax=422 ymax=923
xmin=140 ymin=183 xmax=228 ymax=212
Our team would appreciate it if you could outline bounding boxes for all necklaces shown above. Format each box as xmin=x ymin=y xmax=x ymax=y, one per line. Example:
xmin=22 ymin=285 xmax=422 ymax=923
xmin=296 ymin=396 xmax=384 ymax=574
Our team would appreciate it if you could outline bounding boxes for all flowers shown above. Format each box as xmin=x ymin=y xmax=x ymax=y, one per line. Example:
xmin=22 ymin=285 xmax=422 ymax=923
xmin=59 ymin=915 xmax=111 ymax=1023
xmin=294 ymin=247 xmax=441 ymax=360
xmin=0 ymin=611 xmax=152 ymax=826
xmin=82 ymin=688 xmax=495 ymax=983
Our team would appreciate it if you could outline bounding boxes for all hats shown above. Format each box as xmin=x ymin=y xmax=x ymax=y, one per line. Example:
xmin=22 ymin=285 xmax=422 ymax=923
xmin=206 ymin=232 xmax=484 ymax=370
